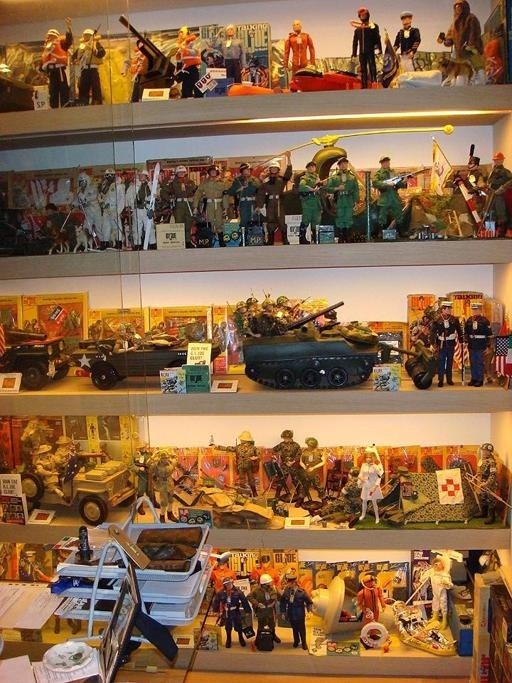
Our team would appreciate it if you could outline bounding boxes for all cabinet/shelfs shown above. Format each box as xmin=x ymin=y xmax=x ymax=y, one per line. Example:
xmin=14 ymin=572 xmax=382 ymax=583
xmin=0 ymin=0 xmax=512 ymax=676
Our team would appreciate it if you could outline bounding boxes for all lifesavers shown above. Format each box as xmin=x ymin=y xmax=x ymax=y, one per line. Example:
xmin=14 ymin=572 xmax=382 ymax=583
xmin=361 ymin=622 xmax=388 ymax=648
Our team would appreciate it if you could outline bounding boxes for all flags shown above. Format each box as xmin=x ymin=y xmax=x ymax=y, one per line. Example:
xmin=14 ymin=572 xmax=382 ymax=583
xmin=431 ymin=143 xmax=454 ymax=196
xmin=493 ymin=334 xmax=512 ymax=377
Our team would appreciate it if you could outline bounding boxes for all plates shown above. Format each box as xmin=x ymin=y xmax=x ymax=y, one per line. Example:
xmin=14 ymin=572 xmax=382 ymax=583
xmin=43 ymin=641 xmax=94 ymax=672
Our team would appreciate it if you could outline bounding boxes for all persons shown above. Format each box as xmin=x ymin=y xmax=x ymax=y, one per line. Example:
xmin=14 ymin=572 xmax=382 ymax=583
xmin=114 ymin=324 xmax=142 ymax=353
xmin=209 ymin=554 xmax=314 ymax=650
xmin=21 ymin=421 xmax=77 ymax=504
xmin=454 ymin=152 xmax=512 ymax=239
xmin=41 ymin=0 xmax=483 ymax=109
xmin=419 ymin=557 xmax=453 ymax=630
xmin=471 ymin=442 xmax=497 ymax=526
xmin=234 ymin=296 xmax=291 ymax=337
xmin=411 ymin=301 xmax=491 ymax=388
xmin=357 ymin=574 xmax=386 ymax=628
xmin=76 ymin=156 xmax=411 ymax=251
xmin=129 ymin=440 xmax=179 ymax=523
xmin=210 ymin=430 xmax=386 ymax=523
xmin=392 ymin=600 xmax=457 ymax=649
